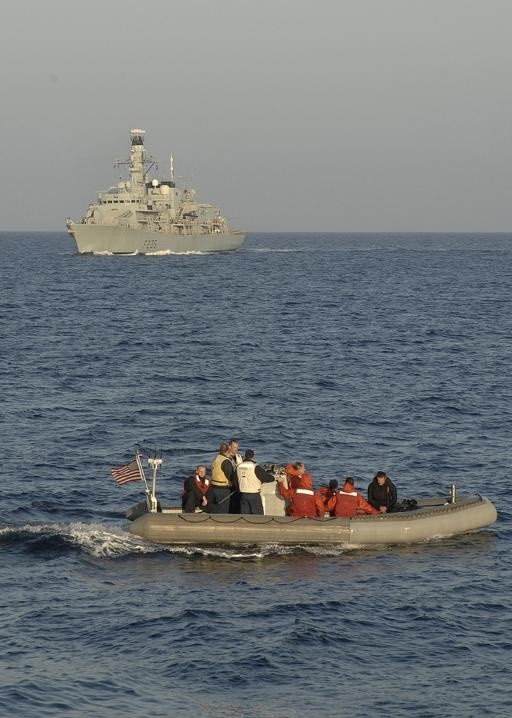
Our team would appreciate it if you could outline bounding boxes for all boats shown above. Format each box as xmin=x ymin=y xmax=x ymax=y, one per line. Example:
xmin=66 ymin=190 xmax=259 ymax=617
xmin=65 ymin=129 xmax=246 ymax=254
xmin=124 ymin=494 xmax=497 ymax=545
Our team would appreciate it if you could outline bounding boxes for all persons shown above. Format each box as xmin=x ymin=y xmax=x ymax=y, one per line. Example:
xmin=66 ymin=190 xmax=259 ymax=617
xmin=181 ymin=439 xmax=397 ymax=517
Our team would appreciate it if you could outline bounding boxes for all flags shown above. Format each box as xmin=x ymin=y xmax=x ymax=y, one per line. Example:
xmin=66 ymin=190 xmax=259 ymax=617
xmin=112 ymin=460 xmax=144 ymax=484
